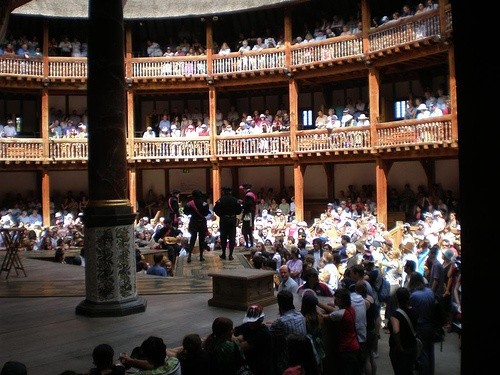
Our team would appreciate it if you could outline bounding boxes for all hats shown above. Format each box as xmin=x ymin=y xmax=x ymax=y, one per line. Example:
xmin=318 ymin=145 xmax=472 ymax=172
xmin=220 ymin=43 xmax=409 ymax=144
xmin=242 ymin=184 xmax=252 ymax=189
xmin=241 ymin=303 xmax=264 ymax=322
xmin=443 ymin=251 xmax=453 ymax=261
xmin=56 ymin=239 xmax=63 ymax=246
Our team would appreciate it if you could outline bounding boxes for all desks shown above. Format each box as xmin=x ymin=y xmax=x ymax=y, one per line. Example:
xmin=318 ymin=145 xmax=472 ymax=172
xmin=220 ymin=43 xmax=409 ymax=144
xmin=0 ymin=228 xmax=26 ymax=279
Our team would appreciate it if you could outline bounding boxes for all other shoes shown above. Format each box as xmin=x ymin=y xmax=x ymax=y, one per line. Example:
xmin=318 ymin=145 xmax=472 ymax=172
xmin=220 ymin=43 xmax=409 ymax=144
xmin=219 ymin=255 xmax=226 ymax=259
xmin=229 ymin=256 xmax=233 ymax=260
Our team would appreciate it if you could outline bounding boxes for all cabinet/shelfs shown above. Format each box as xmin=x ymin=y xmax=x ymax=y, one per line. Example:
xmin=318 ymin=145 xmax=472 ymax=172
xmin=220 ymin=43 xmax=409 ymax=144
xmin=208 ymin=268 xmax=279 ymax=312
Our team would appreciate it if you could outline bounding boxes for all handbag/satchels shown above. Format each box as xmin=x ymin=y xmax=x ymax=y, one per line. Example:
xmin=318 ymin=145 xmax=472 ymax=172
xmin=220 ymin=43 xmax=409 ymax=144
xmin=415 ymin=335 xmax=424 ymax=361
xmin=432 ymin=296 xmax=448 ymax=328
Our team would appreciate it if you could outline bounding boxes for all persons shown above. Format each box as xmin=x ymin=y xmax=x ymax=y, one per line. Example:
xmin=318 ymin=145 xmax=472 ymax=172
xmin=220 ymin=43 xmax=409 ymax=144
xmin=403 ymin=88 xmax=451 ymax=142
xmin=143 ymin=107 xmax=290 ymax=150
xmin=0 ymin=119 xmax=17 ymax=138
xmin=290 ymin=0 xmax=439 ymax=65
xmin=0 ymin=184 xmax=462 ymax=375
xmin=49 ymin=109 xmax=88 ymax=139
xmin=314 ymin=98 xmax=370 ymax=147
xmin=146 ymin=30 xmax=286 ymax=76
xmin=0 ymin=32 xmax=87 ymax=74
